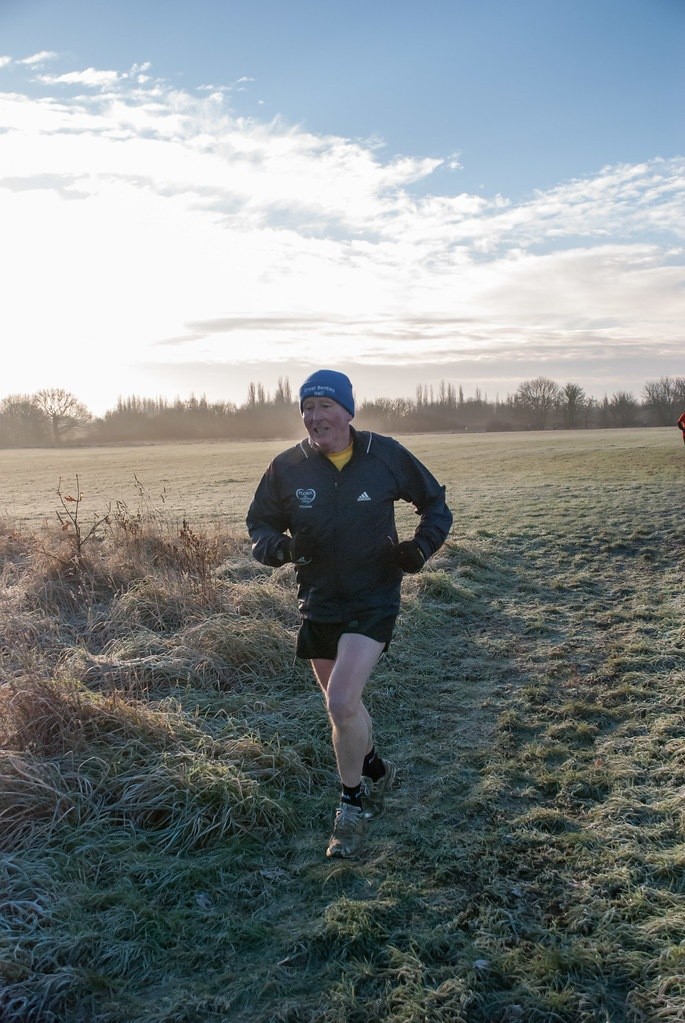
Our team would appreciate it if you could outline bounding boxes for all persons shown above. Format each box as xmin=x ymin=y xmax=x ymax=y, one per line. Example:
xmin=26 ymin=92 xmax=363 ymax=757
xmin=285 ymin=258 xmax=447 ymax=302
xmin=246 ymin=370 xmax=453 ymax=859
xmin=677 ymin=412 xmax=685 ymax=445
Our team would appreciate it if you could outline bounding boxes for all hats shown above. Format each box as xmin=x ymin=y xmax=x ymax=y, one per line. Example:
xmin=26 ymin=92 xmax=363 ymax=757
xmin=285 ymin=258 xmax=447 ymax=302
xmin=298 ymin=369 xmax=354 ymax=418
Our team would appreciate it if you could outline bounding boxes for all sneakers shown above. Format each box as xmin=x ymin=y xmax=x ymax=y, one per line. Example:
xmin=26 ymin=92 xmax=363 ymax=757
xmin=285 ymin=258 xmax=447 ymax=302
xmin=325 ymin=801 xmax=370 ymax=856
xmin=358 ymin=756 xmax=397 ymax=819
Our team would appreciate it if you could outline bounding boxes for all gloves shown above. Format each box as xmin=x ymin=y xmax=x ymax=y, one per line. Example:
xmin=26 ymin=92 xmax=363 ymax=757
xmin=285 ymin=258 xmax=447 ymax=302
xmin=284 ymin=523 xmax=319 ymax=565
xmin=391 ymin=540 xmax=425 ymax=574
